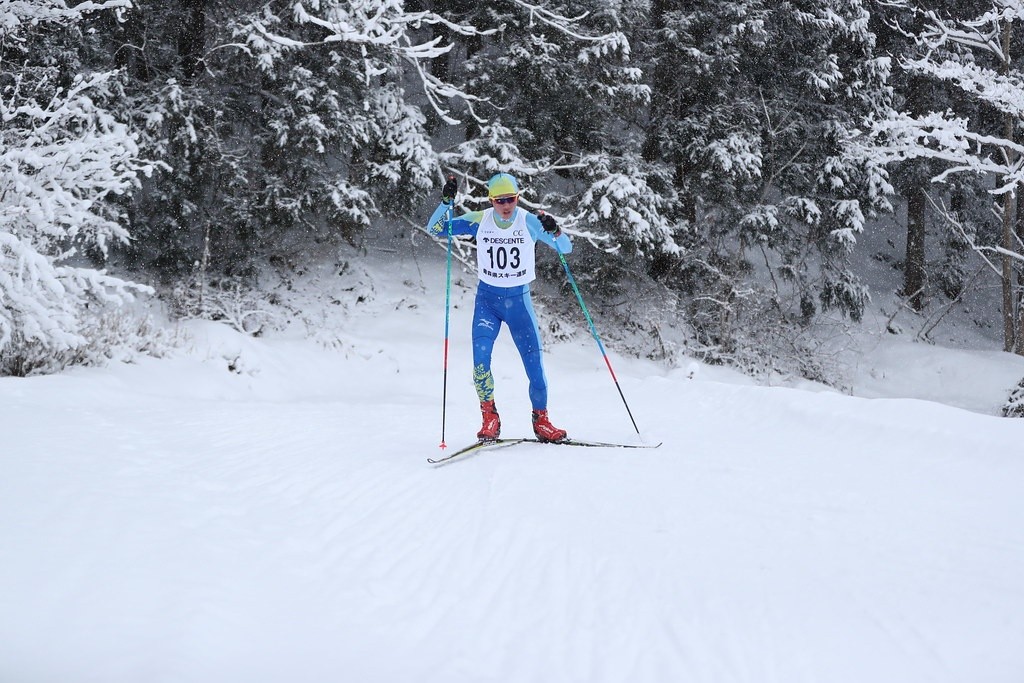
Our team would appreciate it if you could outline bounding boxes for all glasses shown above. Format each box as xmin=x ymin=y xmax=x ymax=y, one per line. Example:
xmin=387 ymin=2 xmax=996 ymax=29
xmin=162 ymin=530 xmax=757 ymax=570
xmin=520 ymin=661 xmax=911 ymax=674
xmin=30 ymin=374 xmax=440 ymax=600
xmin=493 ymin=195 xmax=518 ymax=204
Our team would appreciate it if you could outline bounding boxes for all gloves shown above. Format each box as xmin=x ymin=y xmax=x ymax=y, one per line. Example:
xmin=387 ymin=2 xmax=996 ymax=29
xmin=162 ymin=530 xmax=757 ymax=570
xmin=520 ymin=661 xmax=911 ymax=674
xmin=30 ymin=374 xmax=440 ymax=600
xmin=537 ymin=215 xmax=556 ymax=232
xmin=443 ymin=178 xmax=457 ymax=197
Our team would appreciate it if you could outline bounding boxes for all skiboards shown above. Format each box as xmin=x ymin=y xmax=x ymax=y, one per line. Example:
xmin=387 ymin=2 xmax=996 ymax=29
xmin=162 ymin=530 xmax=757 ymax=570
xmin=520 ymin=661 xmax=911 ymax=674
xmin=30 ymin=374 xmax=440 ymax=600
xmin=427 ymin=436 xmax=663 ymax=464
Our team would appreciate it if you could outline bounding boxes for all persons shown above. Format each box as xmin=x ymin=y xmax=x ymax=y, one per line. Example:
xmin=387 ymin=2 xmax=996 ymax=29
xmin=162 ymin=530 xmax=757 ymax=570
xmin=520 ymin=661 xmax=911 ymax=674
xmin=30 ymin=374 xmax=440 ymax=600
xmin=428 ymin=173 xmax=571 ymax=442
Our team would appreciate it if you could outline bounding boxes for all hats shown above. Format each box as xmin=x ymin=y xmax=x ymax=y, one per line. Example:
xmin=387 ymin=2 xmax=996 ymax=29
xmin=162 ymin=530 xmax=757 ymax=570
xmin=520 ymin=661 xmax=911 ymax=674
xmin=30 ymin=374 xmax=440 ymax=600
xmin=488 ymin=173 xmax=519 ymax=199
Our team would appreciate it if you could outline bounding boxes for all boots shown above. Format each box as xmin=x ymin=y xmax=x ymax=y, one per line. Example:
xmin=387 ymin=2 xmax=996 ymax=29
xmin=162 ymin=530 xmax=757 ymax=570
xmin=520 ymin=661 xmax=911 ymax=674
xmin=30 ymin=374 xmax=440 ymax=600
xmin=532 ymin=408 xmax=566 ymax=441
xmin=478 ymin=399 xmax=501 ymax=437
